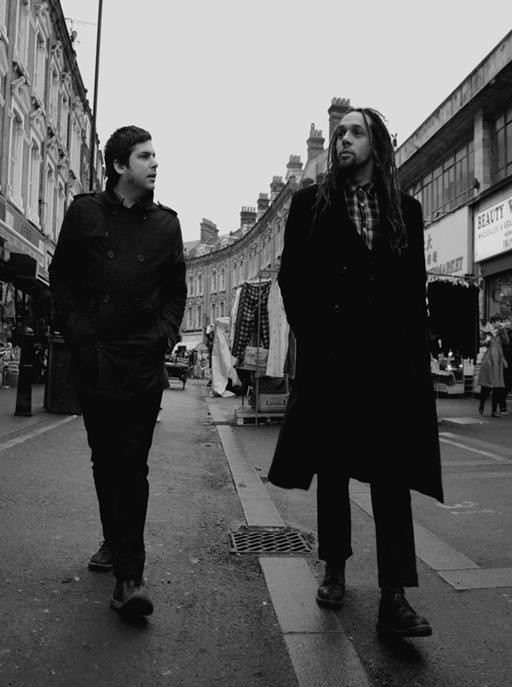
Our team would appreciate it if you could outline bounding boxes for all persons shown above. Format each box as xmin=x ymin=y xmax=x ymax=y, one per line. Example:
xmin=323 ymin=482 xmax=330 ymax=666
xmin=265 ymin=105 xmax=444 ymax=637
xmin=476 ymin=311 xmax=510 ymax=418
xmin=46 ymin=123 xmax=188 ymax=623
xmin=498 ymin=316 xmax=512 ymax=415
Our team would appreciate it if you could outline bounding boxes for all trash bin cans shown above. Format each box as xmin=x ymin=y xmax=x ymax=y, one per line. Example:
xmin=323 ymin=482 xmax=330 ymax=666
xmin=43 ymin=335 xmax=78 ymax=413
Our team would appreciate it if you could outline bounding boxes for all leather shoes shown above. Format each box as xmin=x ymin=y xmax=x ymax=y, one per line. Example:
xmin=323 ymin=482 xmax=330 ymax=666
xmin=86 ymin=538 xmax=115 ymax=571
xmin=316 ymin=572 xmax=346 ymax=609
xmin=376 ymin=591 xmax=432 ymax=636
xmin=110 ymin=578 xmax=155 ymax=617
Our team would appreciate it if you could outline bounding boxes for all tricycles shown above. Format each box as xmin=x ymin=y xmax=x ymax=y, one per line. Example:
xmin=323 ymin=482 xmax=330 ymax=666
xmin=165 ymin=356 xmax=189 ymax=390
